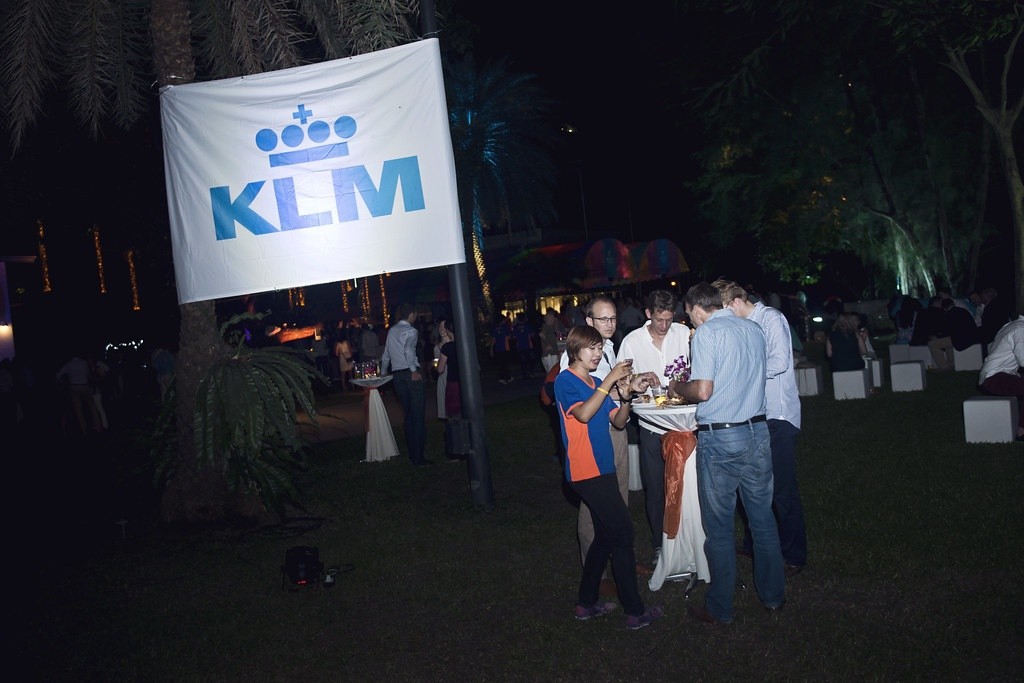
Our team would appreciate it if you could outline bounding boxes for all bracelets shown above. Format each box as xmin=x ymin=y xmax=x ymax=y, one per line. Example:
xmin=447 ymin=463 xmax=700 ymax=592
xmin=597 ymin=387 xmax=608 ymax=395
xmin=622 ymin=397 xmax=632 ymax=402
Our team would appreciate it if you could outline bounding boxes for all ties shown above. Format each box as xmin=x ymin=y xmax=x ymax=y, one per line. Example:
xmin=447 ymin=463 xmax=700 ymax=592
xmin=602 ymin=351 xmax=626 ymax=405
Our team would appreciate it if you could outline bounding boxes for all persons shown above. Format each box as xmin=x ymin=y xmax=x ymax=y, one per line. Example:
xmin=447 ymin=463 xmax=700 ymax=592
xmin=977 ymin=312 xmax=1024 ymax=442
xmin=806 ymin=285 xmax=1009 ymax=372
xmin=555 ymin=276 xmax=805 ymax=629
xmin=305 ymin=302 xmax=469 ymax=466
xmin=0 ymin=345 xmax=175 ymax=436
xmin=486 ymin=306 xmax=561 ymax=382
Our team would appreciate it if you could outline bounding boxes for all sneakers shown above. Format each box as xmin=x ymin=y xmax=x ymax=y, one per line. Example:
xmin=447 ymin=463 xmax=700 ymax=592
xmin=624 ymin=607 xmax=664 ymax=630
xmin=575 ymin=601 xmax=617 ymax=620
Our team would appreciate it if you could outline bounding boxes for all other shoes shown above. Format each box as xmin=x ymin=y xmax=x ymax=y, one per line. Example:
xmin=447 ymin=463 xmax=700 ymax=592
xmin=750 ymin=582 xmax=775 ymax=611
xmin=633 ymin=562 xmax=651 ymax=573
xmin=688 ymin=606 xmax=723 ymax=626
xmin=783 ymin=560 xmax=804 ymax=575
xmin=652 ymin=547 xmax=662 ymax=564
xmin=1015 ymin=432 xmax=1024 ymax=443
xmin=599 ymin=578 xmax=620 ymax=596
xmin=735 ymin=543 xmax=754 ymax=561
xmin=507 ymin=377 xmax=514 ymax=382
xmin=499 ymin=379 xmax=507 ymax=384
xmin=417 ymin=459 xmax=432 ymax=467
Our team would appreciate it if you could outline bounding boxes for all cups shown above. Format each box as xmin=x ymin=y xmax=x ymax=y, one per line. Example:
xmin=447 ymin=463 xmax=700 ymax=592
xmin=651 ymin=386 xmax=668 ymax=405
xmin=353 ymin=359 xmax=382 ymax=379
xmin=432 ymin=357 xmax=440 ymax=367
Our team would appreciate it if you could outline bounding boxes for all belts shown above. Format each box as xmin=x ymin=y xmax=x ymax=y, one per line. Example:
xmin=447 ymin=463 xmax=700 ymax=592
xmin=698 ymin=414 xmax=767 ymax=431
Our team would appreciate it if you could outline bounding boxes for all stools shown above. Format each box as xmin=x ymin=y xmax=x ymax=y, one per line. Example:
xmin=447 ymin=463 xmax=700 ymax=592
xmin=832 ymin=359 xmax=883 ymax=400
xmin=889 ymin=344 xmax=939 ymax=393
xmin=962 ymin=394 xmax=1018 ymax=443
xmin=953 ymin=344 xmax=984 ymax=372
xmin=795 ymin=365 xmax=823 ymax=397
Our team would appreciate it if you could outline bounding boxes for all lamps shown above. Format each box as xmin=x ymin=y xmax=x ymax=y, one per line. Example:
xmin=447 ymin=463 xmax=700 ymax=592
xmin=283 ymin=545 xmax=324 ymax=593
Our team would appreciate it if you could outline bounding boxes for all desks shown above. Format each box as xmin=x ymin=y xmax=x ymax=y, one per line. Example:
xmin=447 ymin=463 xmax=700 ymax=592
xmin=349 ymin=375 xmax=401 ymax=464
xmin=632 ymin=403 xmax=754 ymax=600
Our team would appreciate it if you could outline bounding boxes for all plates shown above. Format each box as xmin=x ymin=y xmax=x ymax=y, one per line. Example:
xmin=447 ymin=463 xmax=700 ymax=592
xmin=665 ymin=401 xmax=697 ymax=408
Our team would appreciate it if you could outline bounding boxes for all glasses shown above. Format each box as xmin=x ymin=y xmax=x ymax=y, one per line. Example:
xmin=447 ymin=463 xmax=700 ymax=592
xmin=587 ymin=315 xmax=617 ymax=323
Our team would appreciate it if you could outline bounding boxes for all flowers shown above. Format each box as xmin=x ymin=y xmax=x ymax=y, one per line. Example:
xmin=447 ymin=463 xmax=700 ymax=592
xmin=663 ymin=355 xmax=693 ymax=383
